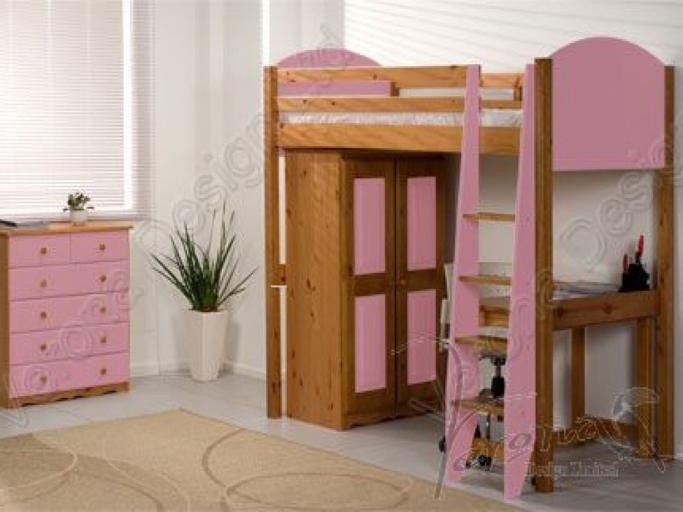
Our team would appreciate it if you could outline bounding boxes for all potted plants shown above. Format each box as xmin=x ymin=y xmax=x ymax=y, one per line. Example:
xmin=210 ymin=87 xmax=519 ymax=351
xmin=63 ymin=192 xmax=96 ymax=226
xmin=142 ymin=202 xmax=259 ymax=384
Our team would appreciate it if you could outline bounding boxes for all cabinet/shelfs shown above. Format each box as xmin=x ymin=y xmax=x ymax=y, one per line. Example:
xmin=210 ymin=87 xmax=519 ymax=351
xmin=0 ymin=222 xmax=133 ymax=410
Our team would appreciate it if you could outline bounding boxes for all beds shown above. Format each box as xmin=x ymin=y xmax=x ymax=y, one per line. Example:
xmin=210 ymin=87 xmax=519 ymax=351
xmin=262 ymin=37 xmax=682 ymax=164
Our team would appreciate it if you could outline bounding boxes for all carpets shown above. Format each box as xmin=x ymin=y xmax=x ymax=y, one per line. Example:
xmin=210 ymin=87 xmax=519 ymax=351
xmin=0 ymin=405 xmax=524 ymax=512
